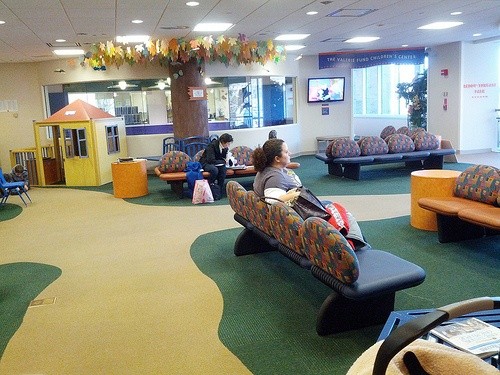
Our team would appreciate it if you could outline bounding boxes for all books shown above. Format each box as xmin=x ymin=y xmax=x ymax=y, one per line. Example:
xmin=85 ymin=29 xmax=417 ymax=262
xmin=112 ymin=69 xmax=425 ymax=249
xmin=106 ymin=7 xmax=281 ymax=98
xmin=429 ymin=316 xmax=500 ymax=358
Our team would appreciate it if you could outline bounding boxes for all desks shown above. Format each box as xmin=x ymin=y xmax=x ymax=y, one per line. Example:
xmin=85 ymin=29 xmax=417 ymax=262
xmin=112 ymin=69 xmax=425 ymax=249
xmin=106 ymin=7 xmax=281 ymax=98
xmin=410 ymin=168 xmax=462 ymax=231
xmin=112 ymin=159 xmax=149 ymax=198
xmin=316 ymin=135 xmax=348 ymax=153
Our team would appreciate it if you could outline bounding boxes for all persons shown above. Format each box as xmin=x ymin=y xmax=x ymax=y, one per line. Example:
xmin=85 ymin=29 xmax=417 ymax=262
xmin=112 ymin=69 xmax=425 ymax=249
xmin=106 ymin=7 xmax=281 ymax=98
xmin=321 ymin=88 xmax=329 ymax=98
xmin=251 ymin=136 xmax=334 ymax=214
xmin=10 ymin=163 xmax=30 ymax=195
xmin=0 ymin=174 xmax=11 ymax=198
xmin=198 ymin=133 xmax=234 ymax=198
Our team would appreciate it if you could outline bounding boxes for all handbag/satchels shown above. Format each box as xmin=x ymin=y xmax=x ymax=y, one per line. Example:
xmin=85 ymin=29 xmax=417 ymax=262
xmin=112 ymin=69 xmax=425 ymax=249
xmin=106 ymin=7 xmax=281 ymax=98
xmin=191 ymin=178 xmax=214 ymax=204
xmin=286 ymin=185 xmax=332 ymax=223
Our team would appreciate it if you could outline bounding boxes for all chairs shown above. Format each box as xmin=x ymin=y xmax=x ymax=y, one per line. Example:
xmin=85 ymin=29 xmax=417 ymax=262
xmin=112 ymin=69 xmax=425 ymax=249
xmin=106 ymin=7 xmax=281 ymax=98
xmin=0 ymin=167 xmax=32 ymax=209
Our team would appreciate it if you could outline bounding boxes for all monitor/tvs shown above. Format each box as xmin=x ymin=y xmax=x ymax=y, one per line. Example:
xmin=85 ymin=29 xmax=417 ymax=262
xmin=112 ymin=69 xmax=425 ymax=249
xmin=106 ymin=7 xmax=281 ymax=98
xmin=307 ymin=77 xmax=345 ymax=103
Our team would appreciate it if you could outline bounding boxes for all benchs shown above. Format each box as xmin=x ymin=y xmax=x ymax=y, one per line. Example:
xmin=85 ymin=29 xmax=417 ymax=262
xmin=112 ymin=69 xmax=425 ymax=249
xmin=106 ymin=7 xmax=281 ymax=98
xmin=418 ymin=165 xmax=500 ymax=239
xmin=224 ymin=181 xmax=426 ymax=336
xmin=314 ymin=125 xmax=455 ymax=181
xmin=153 ymin=146 xmax=301 ymax=193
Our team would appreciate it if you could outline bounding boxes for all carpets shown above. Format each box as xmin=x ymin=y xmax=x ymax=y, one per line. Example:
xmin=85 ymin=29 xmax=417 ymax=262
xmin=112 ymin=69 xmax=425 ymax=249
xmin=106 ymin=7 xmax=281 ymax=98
xmin=190 ymin=214 xmax=500 ymax=375
xmin=0 ymin=262 xmax=63 ymax=358
xmin=0 ymin=203 xmax=23 ymax=222
xmin=33 ymin=153 xmax=471 ymax=206
